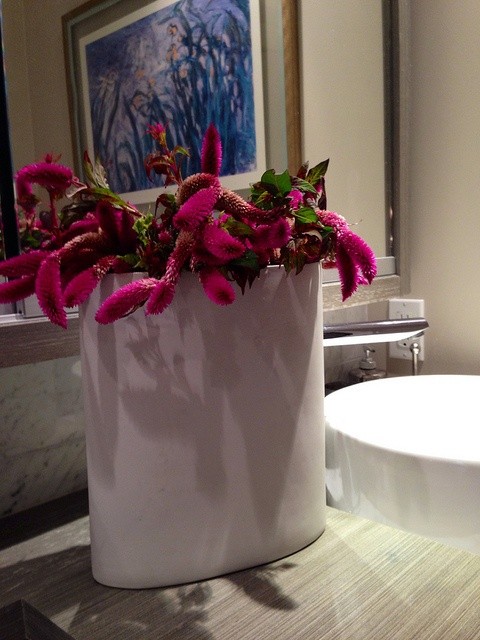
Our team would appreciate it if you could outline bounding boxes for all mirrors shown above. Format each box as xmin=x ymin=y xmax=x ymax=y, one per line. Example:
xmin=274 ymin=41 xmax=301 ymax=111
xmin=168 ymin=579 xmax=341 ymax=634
xmin=0 ymin=0 xmax=397 ymax=327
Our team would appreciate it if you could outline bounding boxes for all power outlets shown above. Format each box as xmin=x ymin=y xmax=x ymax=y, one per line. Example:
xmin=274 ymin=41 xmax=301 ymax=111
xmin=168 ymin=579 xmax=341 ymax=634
xmin=389 ymin=297 xmax=425 ymax=362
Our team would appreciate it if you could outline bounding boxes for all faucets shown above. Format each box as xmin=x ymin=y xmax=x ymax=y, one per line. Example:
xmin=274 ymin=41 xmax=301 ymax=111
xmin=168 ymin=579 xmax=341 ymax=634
xmin=324 ymin=317 xmax=428 ymax=347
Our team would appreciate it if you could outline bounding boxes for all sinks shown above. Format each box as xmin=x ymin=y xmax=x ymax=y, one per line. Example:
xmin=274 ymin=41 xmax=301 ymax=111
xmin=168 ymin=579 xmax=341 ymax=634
xmin=324 ymin=374 xmax=480 ymax=482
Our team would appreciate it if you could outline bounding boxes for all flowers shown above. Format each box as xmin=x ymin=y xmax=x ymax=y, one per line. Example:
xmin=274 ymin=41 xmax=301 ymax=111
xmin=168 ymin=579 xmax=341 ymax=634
xmin=2 ymin=123 xmax=379 ymax=323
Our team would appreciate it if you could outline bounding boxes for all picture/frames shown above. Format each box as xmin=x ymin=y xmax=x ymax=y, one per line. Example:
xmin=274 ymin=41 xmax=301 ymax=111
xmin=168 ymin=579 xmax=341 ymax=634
xmin=61 ymin=0 xmax=301 ymax=232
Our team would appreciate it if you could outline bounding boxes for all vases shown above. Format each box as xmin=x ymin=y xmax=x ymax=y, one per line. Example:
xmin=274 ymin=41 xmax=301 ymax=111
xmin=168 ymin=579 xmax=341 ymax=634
xmin=77 ymin=257 xmax=327 ymax=592
